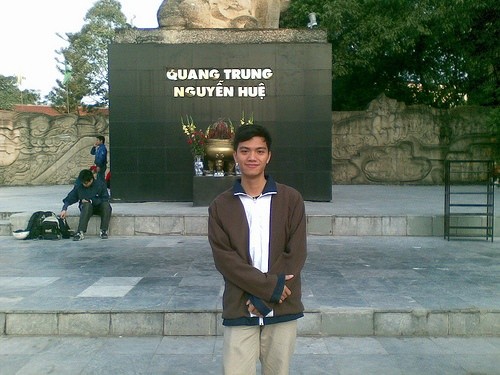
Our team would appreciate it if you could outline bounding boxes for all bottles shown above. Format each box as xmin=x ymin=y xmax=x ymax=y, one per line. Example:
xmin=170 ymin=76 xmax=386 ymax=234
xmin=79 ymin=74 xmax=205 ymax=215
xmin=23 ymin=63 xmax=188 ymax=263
xmin=194 ymin=154 xmax=204 ymax=175
xmin=235 ymin=162 xmax=242 ymax=176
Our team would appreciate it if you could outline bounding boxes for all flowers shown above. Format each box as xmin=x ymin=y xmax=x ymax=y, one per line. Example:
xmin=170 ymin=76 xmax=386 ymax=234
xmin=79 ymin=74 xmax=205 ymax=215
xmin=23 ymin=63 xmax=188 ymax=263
xmin=181 ymin=113 xmax=210 ymax=159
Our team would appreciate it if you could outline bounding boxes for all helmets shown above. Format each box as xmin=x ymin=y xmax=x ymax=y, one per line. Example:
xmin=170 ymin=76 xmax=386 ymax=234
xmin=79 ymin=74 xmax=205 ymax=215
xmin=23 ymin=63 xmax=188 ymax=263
xmin=12 ymin=229 xmax=30 ymax=240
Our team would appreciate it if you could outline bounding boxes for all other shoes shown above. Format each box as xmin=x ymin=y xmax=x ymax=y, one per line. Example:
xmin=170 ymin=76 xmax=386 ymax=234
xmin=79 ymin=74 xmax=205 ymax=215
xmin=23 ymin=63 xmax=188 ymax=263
xmin=72 ymin=230 xmax=84 ymax=241
xmin=100 ymin=229 xmax=109 ymax=239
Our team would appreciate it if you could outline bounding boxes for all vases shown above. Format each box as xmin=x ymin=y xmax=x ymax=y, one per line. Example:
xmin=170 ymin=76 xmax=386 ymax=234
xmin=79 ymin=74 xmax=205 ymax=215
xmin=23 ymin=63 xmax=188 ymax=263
xmin=195 ymin=155 xmax=204 ymax=176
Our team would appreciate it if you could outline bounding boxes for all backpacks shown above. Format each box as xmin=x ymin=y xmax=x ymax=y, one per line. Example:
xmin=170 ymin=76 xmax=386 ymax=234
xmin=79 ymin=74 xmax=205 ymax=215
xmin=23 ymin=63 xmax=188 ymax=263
xmin=26 ymin=211 xmax=71 ymax=240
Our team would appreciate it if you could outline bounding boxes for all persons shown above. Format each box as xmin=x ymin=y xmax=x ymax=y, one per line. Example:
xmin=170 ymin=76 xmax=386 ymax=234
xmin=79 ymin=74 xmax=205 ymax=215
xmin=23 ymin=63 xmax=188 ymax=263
xmin=207 ymin=125 xmax=308 ymax=375
xmin=58 ymin=169 xmax=112 ymax=241
xmin=90 ymin=136 xmax=107 ymax=182
